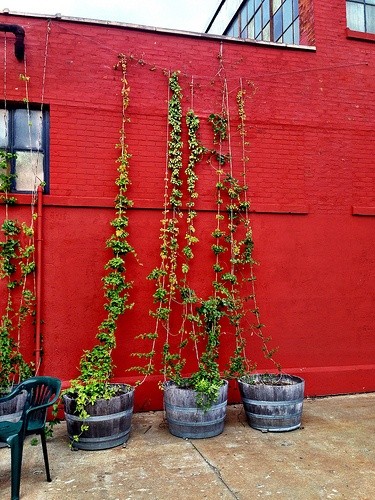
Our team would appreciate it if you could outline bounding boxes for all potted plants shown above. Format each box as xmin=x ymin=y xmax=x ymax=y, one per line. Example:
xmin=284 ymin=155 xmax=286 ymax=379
xmin=164 ymin=68 xmax=232 ymax=440
xmin=236 ymin=75 xmax=306 ymax=433
xmin=62 ymin=53 xmax=136 ymax=451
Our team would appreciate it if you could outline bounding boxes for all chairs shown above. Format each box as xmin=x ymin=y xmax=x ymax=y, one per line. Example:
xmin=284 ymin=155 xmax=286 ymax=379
xmin=1 ymin=375 xmax=61 ymax=500
